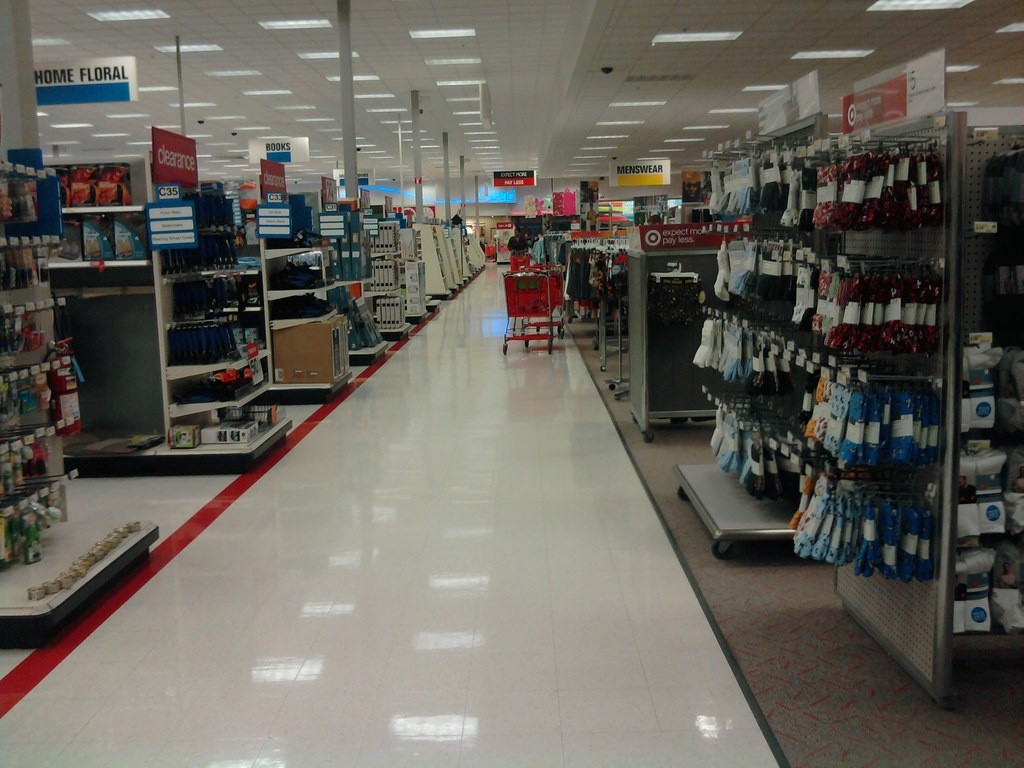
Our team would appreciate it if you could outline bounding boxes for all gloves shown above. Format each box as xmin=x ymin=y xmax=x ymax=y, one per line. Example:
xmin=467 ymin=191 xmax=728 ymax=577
xmin=693 ymin=150 xmax=814 ymax=502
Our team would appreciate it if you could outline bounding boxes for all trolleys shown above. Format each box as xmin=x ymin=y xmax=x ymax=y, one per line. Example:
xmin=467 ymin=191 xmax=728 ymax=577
xmin=510 ymin=251 xmax=531 ymax=275
xmin=484 ymin=244 xmax=498 ymax=264
xmin=501 ymin=264 xmax=565 ymax=356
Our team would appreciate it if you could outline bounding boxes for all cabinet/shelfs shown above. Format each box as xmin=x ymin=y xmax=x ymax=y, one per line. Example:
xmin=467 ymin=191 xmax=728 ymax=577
xmin=413 ymin=222 xmax=486 ymax=313
xmin=318 ymin=190 xmax=389 ymax=367
xmin=357 ymin=198 xmax=412 ymax=342
xmin=0 ymin=156 xmax=353 ymax=651
xmin=382 ymin=204 xmax=427 ymax=325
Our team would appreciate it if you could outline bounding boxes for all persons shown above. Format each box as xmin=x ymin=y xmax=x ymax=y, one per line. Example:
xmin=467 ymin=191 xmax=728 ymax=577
xmin=586 ymin=202 xmax=599 ymax=232
xmin=646 ymin=214 xmax=663 ymax=226
xmin=507 ymin=227 xmax=528 ymax=256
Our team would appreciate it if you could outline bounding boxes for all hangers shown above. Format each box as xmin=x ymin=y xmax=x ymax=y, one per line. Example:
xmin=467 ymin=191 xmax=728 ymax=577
xmin=544 ymin=229 xmax=629 ymax=254
xmin=651 ymin=261 xmax=698 ymax=284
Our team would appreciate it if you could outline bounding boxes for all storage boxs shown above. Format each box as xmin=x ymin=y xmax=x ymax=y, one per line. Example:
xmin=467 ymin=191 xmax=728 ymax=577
xmin=81 ymin=213 xmax=116 ymax=261
xmin=67 ymin=163 xmax=97 ymax=207
xmin=201 ymin=420 xmax=259 ymax=444
xmin=325 ymin=208 xmax=427 ymax=351
xmin=272 ymin=317 xmax=345 ymax=383
xmin=43 ymin=165 xmax=71 ymax=207
xmin=332 ymin=315 xmax=350 ymax=374
xmin=166 ymin=423 xmax=202 ymax=450
xmin=97 ymin=162 xmax=132 ymax=205
xmin=50 ymin=220 xmax=82 ymax=263
xmin=112 ymin=212 xmax=150 ymax=260
